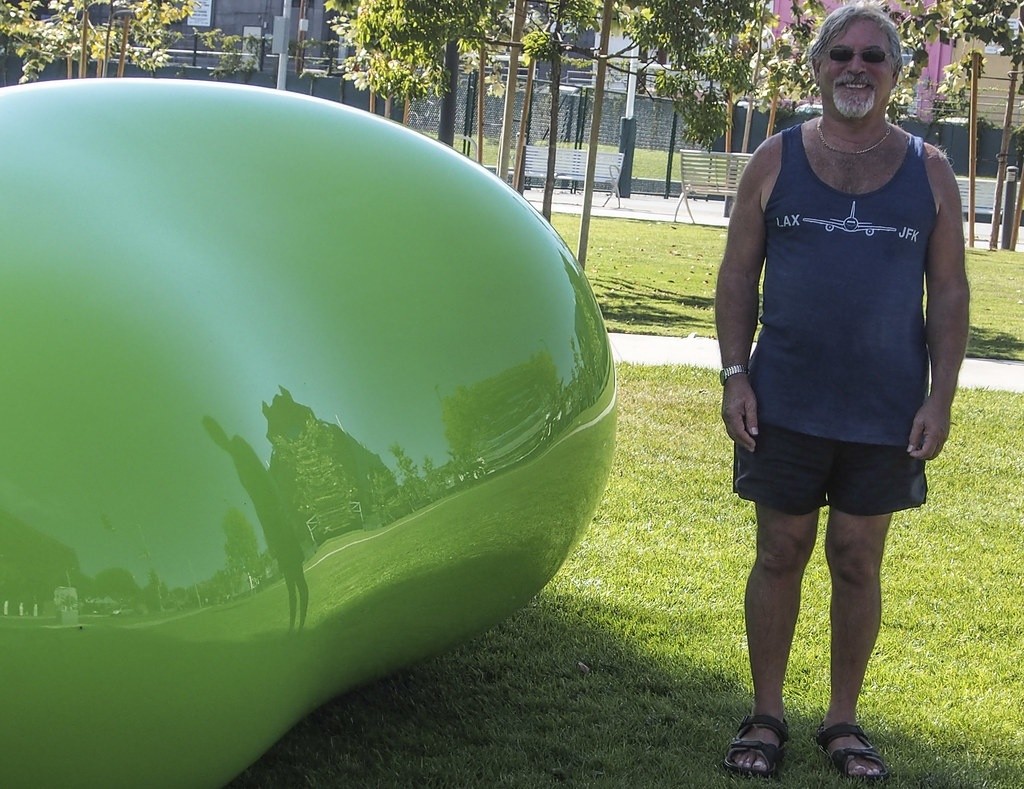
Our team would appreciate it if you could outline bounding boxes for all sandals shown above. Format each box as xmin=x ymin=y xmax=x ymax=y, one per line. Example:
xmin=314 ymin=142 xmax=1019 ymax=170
xmin=814 ymin=719 xmax=890 ymax=780
xmin=723 ymin=712 xmax=790 ymax=776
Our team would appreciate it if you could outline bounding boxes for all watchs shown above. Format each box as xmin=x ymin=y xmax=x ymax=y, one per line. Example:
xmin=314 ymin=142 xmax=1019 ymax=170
xmin=719 ymin=364 xmax=747 ymax=386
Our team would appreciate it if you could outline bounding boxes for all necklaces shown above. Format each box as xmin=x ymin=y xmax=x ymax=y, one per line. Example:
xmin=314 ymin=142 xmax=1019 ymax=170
xmin=816 ymin=118 xmax=891 ymax=155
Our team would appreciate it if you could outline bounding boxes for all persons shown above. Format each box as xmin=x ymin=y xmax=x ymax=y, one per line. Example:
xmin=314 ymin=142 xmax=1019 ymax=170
xmin=202 ymin=413 xmax=310 ymax=639
xmin=712 ymin=8 xmax=971 ymax=778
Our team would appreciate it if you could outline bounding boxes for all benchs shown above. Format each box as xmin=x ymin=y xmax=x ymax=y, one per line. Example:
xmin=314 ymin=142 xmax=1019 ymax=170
xmin=950 ymin=178 xmax=1021 ymax=235
xmin=521 ymin=145 xmax=625 ymax=209
xmin=673 ymin=149 xmax=758 ymax=225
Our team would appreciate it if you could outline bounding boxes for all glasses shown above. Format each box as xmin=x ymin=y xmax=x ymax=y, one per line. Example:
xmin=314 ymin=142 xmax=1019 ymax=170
xmin=822 ymin=47 xmax=894 ymax=63
